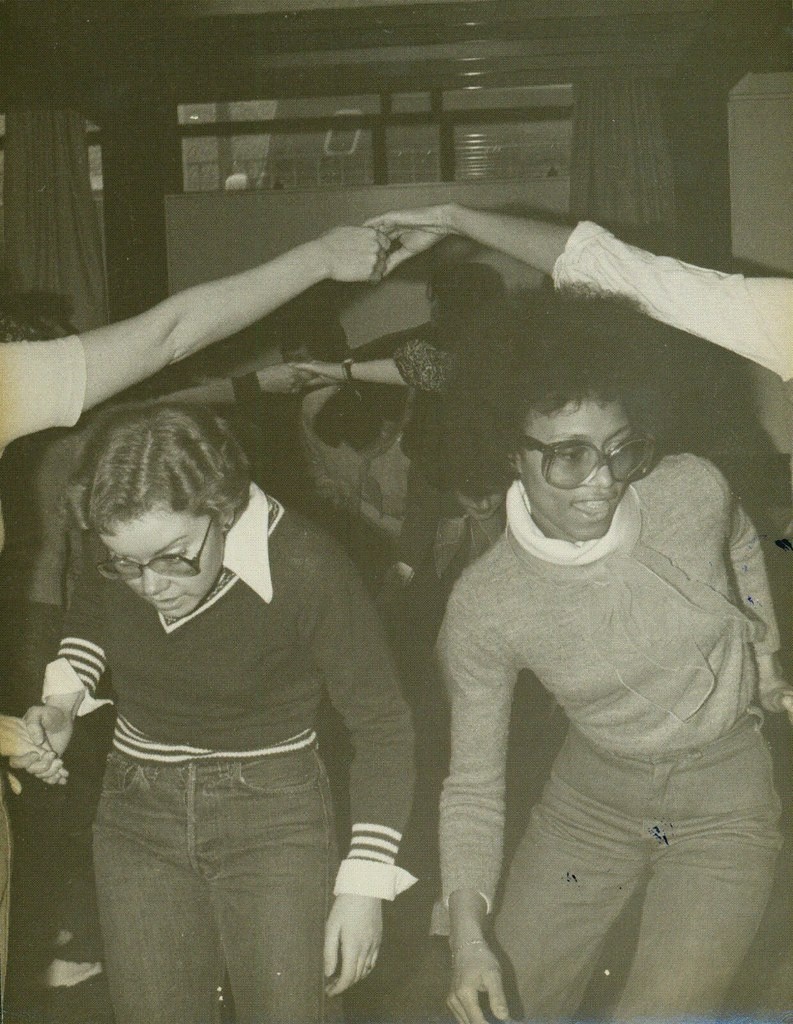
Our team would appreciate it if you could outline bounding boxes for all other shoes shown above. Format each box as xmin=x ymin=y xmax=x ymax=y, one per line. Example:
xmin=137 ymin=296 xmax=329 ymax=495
xmin=24 ymin=956 xmax=101 ymax=993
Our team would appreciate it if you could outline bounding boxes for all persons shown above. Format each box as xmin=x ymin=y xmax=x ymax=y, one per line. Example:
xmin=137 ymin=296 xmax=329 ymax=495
xmin=0 ymin=287 xmax=326 ymax=1024
xmin=0 ymin=712 xmax=70 ymax=797
xmin=266 ymin=315 xmax=360 ymax=504
xmin=288 ymin=260 xmax=542 ymax=789
xmin=434 ymin=341 xmax=793 ymax=1022
xmin=11 ymin=398 xmax=417 ymax=1022
xmin=364 ymin=204 xmax=793 ymax=392
xmin=0 ymin=221 xmax=391 ymax=449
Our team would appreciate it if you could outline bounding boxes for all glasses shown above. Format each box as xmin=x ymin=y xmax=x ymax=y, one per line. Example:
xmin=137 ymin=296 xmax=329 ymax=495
xmin=95 ymin=514 xmax=214 ymax=580
xmin=515 ymin=430 xmax=657 ymax=489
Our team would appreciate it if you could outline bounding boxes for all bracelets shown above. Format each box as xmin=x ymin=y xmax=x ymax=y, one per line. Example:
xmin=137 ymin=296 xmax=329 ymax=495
xmin=232 ymin=371 xmax=262 ymax=405
xmin=342 ymin=357 xmax=354 ymax=381
